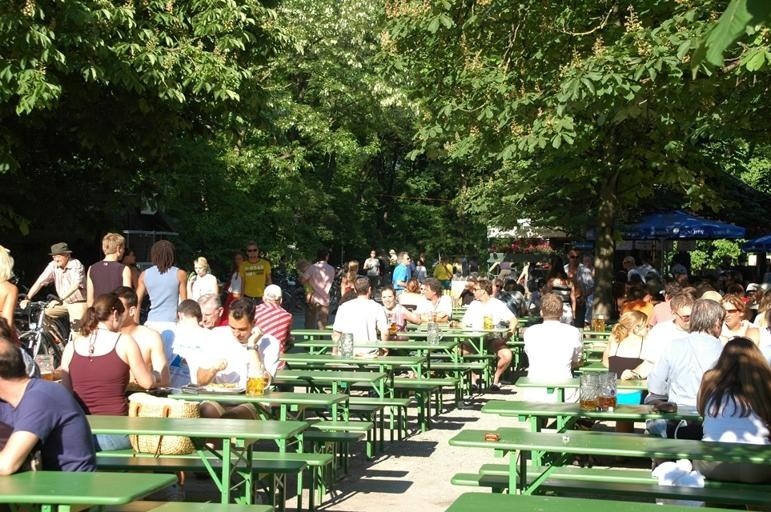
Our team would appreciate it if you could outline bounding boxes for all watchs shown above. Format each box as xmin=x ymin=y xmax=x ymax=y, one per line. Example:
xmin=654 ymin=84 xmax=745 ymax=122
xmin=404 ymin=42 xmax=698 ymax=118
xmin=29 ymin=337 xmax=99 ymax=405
xmin=246 ymin=345 xmax=261 ymax=352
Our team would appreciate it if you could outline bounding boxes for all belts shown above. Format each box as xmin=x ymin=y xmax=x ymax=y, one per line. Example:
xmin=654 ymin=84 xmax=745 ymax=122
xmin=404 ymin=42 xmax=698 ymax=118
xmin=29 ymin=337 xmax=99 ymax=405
xmin=244 ymin=294 xmax=263 ymax=301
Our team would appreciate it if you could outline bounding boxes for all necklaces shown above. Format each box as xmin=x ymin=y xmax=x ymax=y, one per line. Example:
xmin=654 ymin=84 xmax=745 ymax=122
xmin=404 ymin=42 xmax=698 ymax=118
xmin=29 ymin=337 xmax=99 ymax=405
xmin=88 ymin=328 xmax=112 ymax=354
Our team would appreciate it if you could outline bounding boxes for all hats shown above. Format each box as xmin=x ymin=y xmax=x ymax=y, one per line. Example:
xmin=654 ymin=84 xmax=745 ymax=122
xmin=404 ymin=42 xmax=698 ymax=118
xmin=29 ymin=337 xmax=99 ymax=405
xmin=746 ymin=283 xmax=759 ymax=293
xmin=47 ymin=242 xmax=72 ymax=254
xmin=701 ymin=291 xmax=723 ymax=304
xmin=761 ymin=283 xmax=771 ymax=295
xmin=389 ymin=249 xmax=396 ymax=254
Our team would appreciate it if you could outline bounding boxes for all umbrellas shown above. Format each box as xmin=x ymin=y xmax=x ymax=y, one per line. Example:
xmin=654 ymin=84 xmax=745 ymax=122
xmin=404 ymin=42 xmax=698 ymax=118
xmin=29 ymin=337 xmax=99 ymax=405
xmin=622 ymin=207 xmax=745 ymax=263
xmin=742 ymin=229 xmax=770 ymax=254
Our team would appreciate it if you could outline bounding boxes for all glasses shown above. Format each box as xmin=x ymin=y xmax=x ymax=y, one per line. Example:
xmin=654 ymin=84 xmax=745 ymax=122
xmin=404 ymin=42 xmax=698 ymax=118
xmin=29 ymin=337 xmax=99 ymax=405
xmin=726 ymin=309 xmax=735 ymax=314
xmin=676 ymin=312 xmax=689 ymax=322
xmin=569 ymin=254 xmax=579 ymax=259
xmin=472 ymin=288 xmax=482 ymax=292
xmin=624 ymin=261 xmax=630 ymax=263
xmin=495 ymin=285 xmax=502 ymax=288
xmin=246 ymin=248 xmax=257 ymax=252
xmin=404 ymin=258 xmax=411 ymax=260
xmin=748 ymin=293 xmax=756 ymax=297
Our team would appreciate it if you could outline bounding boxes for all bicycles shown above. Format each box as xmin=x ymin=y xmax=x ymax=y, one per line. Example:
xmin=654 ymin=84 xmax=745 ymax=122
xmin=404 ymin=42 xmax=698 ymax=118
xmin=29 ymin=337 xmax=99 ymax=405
xmin=16 ymin=301 xmax=65 ymax=370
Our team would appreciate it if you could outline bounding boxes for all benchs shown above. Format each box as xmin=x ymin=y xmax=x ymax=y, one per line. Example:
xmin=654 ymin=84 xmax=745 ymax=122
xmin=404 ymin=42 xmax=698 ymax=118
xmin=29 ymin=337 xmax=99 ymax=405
xmin=454 ymin=474 xmax=655 ymax=498
xmin=102 ymin=500 xmax=273 ymax=512
xmin=97 ymin=451 xmax=333 ymax=504
xmin=270 ymin=377 xmax=457 ymax=434
xmin=339 ymin=396 xmax=411 ymax=444
xmin=480 ymin=464 xmax=653 ymax=479
xmin=300 ymin=432 xmax=363 ymax=475
xmin=445 ymin=491 xmax=771 ymax=511
xmin=95 ymin=460 xmax=307 ymax=506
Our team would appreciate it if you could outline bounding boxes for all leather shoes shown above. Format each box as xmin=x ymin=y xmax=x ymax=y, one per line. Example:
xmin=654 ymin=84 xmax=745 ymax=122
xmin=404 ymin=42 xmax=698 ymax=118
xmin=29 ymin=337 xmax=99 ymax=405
xmin=491 ymin=384 xmax=499 ymax=391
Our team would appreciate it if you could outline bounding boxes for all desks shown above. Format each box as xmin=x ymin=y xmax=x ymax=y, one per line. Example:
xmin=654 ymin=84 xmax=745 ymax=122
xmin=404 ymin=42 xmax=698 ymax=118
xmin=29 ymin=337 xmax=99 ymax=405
xmin=1 ymin=470 xmax=178 ymax=512
xmin=85 ymin=415 xmax=309 ymax=511
xmin=518 ymin=377 xmax=649 ymax=401
xmin=168 ymin=393 xmax=349 ymax=489
xmin=576 ymin=329 xmax=618 ymax=374
xmin=484 ymin=400 xmax=698 ymax=433
xmin=280 ymin=307 xmax=539 ymax=386
xmin=277 ymin=370 xmax=386 ymax=449
xmin=452 ymin=430 xmax=771 ymax=496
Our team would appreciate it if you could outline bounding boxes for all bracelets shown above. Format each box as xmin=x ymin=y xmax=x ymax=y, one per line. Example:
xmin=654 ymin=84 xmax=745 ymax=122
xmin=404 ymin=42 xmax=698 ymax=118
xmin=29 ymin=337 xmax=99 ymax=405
xmin=23 ymin=295 xmax=31 ymax=301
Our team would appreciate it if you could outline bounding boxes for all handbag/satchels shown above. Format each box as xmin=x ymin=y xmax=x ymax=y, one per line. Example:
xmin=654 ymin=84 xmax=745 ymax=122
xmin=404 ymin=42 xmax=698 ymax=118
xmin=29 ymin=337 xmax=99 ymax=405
xmin=0 ymin=422 xmax=44 ymax=511
xmin=127 ymin=393 xmax=200 ymax=454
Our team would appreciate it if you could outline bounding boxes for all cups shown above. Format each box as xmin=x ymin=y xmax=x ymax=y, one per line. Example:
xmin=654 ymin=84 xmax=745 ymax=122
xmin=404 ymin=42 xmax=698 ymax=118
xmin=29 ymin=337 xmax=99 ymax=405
xmin=595 ymin=314 xmax=604 ymax=331
xmin=246 ymin=362 xmax=271 ymax=397
xmin=340 ymin=333 xmax=354 ymax=359
xmin=578 ymin=373 xmax=600 ymax=411
xmin=600 ymin=370 xmax=616 ymax=408
xmin=483 ymin=314 xmax=493 ymax=330
xmin=35 ymin=354 xmax=55 ymax=382
xmin=427 ymin=323 xmax=444 ymax=346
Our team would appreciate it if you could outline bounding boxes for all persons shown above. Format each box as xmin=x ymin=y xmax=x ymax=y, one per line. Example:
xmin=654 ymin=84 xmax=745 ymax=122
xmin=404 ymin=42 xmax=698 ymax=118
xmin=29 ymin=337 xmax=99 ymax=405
xmin=414 ymin=277 xmax=452 ymax=323
xmin=524 ymin=293 xmax=595 ymax=466
xmin=331 ymin=275 xmax=389 ymax=358
xmin=239 ymin=240 xmax=271 ymax=304
xmin=492 ymin=277 xmax=525 ymax=319
xmin=377 ymin=249 xmax=391 ymax=270
xmin=717 ymin=293 xmax=760 ymax=346
xmin=61 ymin=295 xmax=161 ymax=452
xmin=254 ymin=284 xmax=295 ymax=371
xmin=388 ymin=249 xmax=398 ymax=267
xmin=0 ymin=340 xmax=95 ymax=475
xmin=119 ymin=247 xmax=148 ymax=296
xmin=646 ymin=299 xmax=724 ymax=440
xmin=223 ymin=252 xmax=243 ymax=303
xmin=612 ymin=255 xmax=665 ymax=310
xmin=696 ymin=336 xmax=771 ymax=445
xmin=391 ymin=251 xmax=411 ymax=298
xmin=159 ymin=299 xmax=211 ymax=387
xmin=526 ymin=280 xmax=541 ymax=315
xmin=133 ymin=240 xmax=186 ymax=334
xmin=20 ymin=242 xmax=86 ymax=338
xmin=620 ymin=294 xmax=697 ymax=439
xmin=544 ymin=256 xmax=576 ymax=327
xmin=665 ymin=264 xmax=727 ymax=300
xmin=196 ymin=293 xmax=228 ymax=330
xmin=297 ymin=261 xmax=314 ymax=303
xmin=410 ymin=256 xmax=416 ymax=271
xmin=431 ymin=255 xmax=479 ymax=305
xmin=341 ymin=260 xmax=361 ymax=297
xmin=0 ymin=248 xmax=18 ymax=326
xmin=86 ymin=233 xmax=132 ymax=307
xmin=195 ymin=298 xmax=281 ymax=450
xmin=564 ymin=248 xmax=594 ymax=328
xmin=582 ymin=251 xmax=595 ymax=323
xmin=603 ymin=310 xmax=648 ymax=380
xmin=414 ymin=260 xmax=428 ymax=277
xmin=753 ymin=289 xmax=771 ymax=329
xmin=54 ymin=287 xmax=169 ymax=389
xmin=185 ymin=256 xmax=218 ymax=300
xmin=398 ymin=278 xmax=423 ymax=307
xmin=363 ymin=250 xmax=380 ymax=300
xmin=727 ymin=270 xmax=745 ymax=293
xmin=448 ymin=279 xmax=517 ymax=391
xmin=380 ymin=285 xmax=422 ymax=332
xmin=0 ymin=315 xmax=41 ymax=378
xmin=300 ymin=246 xmax=335 ymax=351
xmin=744 ymin=283 xmax=760 ymax=321
xmin=761 ymin=282 xmax=771 ymax=296
xmin=726 ymin=282 xmax=749 ymax=304
